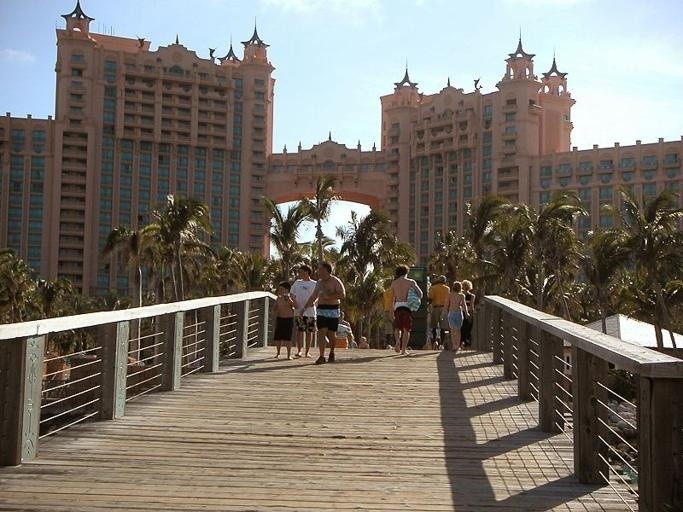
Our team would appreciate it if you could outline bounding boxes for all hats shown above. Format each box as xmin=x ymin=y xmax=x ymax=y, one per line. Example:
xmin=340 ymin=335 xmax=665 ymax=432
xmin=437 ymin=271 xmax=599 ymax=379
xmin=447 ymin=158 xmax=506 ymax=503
xmin=438 ymin=275 xmax=446 ymax=283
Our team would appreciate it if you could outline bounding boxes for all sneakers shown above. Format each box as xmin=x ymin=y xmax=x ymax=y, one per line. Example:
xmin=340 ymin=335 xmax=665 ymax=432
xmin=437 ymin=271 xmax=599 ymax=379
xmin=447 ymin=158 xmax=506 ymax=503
xmin=316 ymin=353 xmax=335 ymax=364
xmin=433 ymin=337 xmax=470 ymax=351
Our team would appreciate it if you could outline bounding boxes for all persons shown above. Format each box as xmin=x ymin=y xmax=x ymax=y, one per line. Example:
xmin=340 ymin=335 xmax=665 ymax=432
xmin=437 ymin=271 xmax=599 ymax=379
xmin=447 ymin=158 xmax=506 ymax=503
xmin=335 ymin=263 xmax=476 ymax=349
xmin=300 ymin=263 xmax=346 ymax=364
xmin=273 ymin=280 xmax=297 ymax=359
xmin=290 ymin=265 xmax=318 ymax=358
